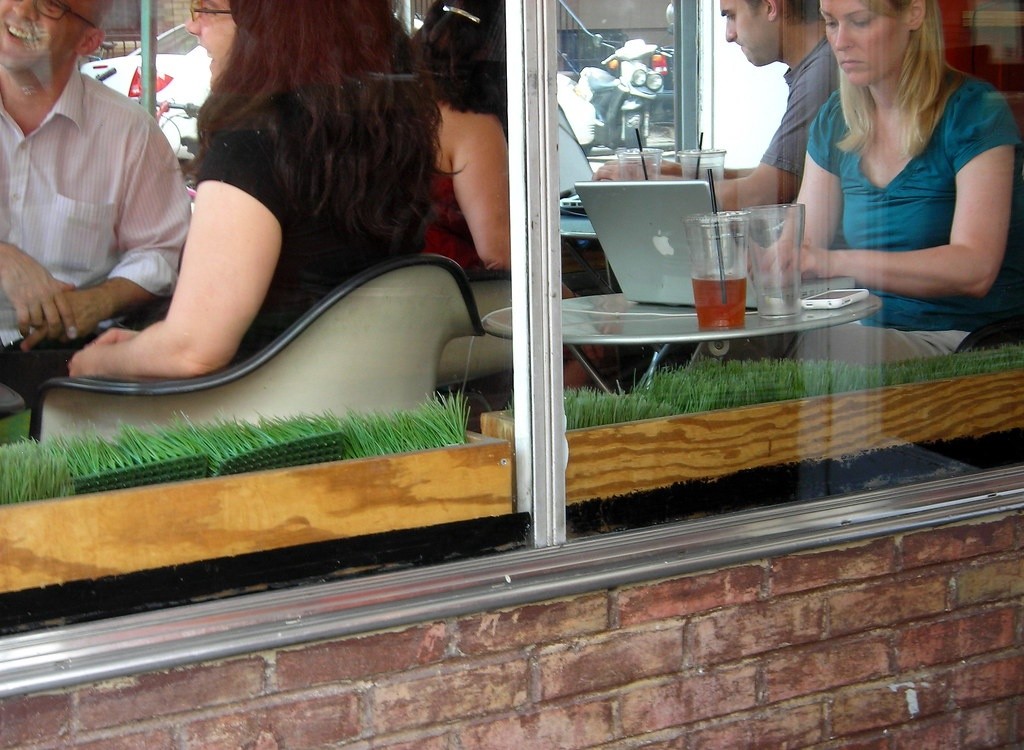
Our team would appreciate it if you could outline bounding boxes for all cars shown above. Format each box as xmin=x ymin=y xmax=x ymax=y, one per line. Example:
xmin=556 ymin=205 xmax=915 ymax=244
xmin=79 ymin=22 xmax=216 ymax=167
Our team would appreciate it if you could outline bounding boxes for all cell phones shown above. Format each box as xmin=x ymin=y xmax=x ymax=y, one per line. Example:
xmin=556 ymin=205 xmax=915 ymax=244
xmin=802 ymin=289 xmax=869 ymax=310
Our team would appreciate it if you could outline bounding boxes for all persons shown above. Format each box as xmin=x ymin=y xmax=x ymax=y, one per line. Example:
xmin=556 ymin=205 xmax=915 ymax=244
xmin=405 ymin=1 xmax=594 ymax=397
xmin=0 ymin=0 xmax=191 ymax=370
xmin=730 ymin=0 xmax=1019 ymax=372
xmin=68 ymin=0 xmax=468 ymax=384
xmin=583 ymin=1 xmax=842 ymax=209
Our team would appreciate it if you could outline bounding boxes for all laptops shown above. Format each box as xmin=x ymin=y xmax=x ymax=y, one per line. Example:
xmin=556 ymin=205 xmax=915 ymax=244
xmin=574 ymin=180 xmax=829 ymax=309
xmin=558 ymin=105 xmax=597 ymax=216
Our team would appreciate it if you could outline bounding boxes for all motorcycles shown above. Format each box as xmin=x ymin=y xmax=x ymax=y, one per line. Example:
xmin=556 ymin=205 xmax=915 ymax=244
xmin=576 ymin=34 xmax=674 ymax=150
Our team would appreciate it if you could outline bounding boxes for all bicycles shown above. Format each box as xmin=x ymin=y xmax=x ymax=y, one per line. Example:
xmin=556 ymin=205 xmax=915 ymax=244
xmin=96 ymin=68 xmax=204 ymax=164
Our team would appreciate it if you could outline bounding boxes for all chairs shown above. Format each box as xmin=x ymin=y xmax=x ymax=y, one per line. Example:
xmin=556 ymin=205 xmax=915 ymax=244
xmin=22 ymin=253 xmax=490 ymax=447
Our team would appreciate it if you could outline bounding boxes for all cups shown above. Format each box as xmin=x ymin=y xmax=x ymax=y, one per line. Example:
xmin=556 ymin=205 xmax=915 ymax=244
xmin=676 ymin=149 xmax=728 ymax=181
xmin=681 ymin=210 xmax=752 ymax=328
xmin=742 ymin=203 xmax=806 ymax=319
xmin=618 ymin=148 xmax=664 ymax=182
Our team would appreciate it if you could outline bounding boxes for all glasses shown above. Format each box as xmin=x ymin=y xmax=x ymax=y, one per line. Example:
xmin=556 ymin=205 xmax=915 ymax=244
xmin=32 ymin=0 xmax=98 ymax=32
xmin=191 ymin=0 xmax=232 ymax=22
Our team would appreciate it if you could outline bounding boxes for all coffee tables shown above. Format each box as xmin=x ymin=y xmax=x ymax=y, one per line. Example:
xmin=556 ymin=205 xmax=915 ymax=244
xmin=475 ymin=291 xmax=883 ymax=377
xmin=556 ymin=193 xmax=615 ymax=293
